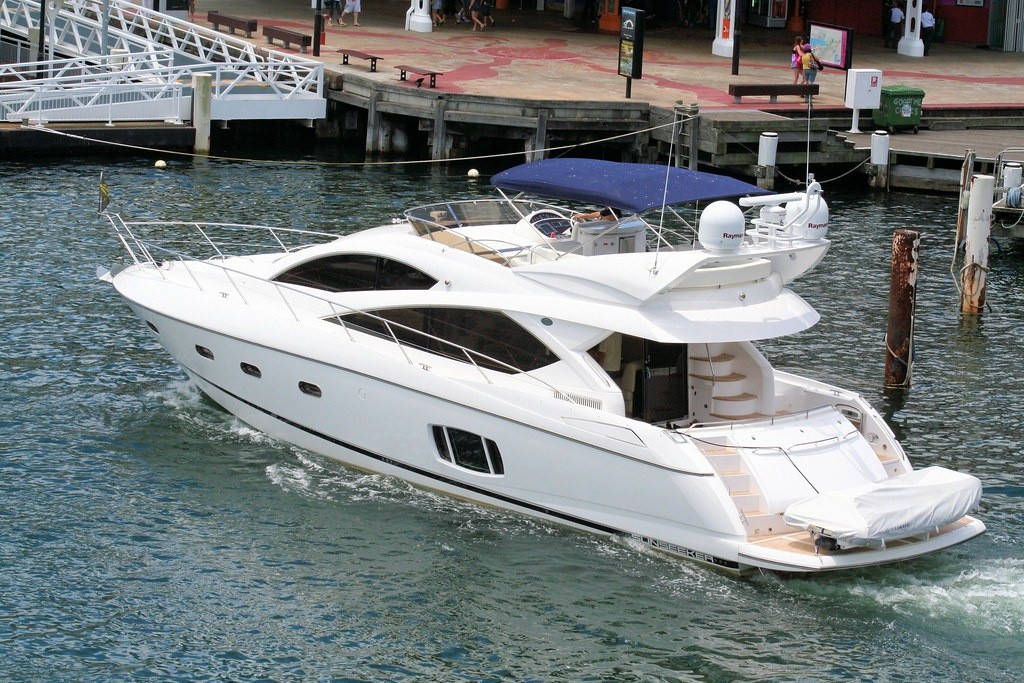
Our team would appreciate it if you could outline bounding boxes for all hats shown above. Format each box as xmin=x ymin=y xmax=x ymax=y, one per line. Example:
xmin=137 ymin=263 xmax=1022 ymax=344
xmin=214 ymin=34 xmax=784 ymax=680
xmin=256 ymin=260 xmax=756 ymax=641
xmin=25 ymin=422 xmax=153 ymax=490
xmin=803 ymin=44 xmax=811 ymax=51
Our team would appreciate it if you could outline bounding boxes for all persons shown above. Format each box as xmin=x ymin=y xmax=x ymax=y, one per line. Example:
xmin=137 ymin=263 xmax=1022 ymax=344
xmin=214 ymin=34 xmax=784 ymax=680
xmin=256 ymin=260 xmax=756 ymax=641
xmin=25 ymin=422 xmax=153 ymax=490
xmin=482 ymin=0 xmax=495 ymax=27
xmin=921 ymin=6 xmax=935 ymax=56
xmin=800 ymin=44 xmax=820 ymax=98
xmin=430 ymin=0 xmax=447 ymax=27
xmin=790 ymin=36 xmax=805 ymax=84
xmin=324 ymin=0 xmax=347 ymax=27
xmin=598 ymin=332 xmax=622 ymax=379
xmin=454 ymin=0 xmax=470 ymax=22
xmin=468 ymin=0 xmax=486 ymax=32
xmin=884 ymin=3 xmax=905 ymax=49
xmin=338 ymin=0 xmax=361 ymax=26
xmin=576 ymin=207 xmax=622 ymax=221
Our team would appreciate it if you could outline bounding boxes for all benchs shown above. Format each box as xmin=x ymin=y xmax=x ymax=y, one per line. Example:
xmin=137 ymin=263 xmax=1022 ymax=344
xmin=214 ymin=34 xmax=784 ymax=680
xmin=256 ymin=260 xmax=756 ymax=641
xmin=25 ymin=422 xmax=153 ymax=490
xmin=263 ymin=25 xmax=312 ymax=53
xmin=335 ymin=50 xmax=383 ymax=72
xmin=208 ymin=11 xmax=257 ymax=38
xmin=394 ymin=65 xmax=443 ymax=88
xmin=729 ymin=83 xmax=819 ymax=105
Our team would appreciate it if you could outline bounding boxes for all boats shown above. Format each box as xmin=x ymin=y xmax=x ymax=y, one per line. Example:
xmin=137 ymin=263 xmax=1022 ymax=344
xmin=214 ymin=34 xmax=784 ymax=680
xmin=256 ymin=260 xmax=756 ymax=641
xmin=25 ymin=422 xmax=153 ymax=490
xmin=92 ymin=101 xmax=983 ymax=578
xmin=992 ymin=147 xmax=1024 ymax=227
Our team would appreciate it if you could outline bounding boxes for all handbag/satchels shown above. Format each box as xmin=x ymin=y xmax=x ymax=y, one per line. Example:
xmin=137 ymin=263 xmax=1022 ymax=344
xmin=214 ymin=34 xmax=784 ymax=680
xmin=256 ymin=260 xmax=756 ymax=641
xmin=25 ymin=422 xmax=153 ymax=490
xmin=808 ymin=59 xmax=823 ymax=71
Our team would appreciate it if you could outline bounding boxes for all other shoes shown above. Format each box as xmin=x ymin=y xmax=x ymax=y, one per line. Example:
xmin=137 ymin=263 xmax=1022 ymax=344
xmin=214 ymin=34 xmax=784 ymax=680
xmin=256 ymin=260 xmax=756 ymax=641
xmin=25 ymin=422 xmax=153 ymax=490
xmin=439 ymin=20 xmax=443 ymax=26
xmin=470 ymin=29 xmax=476 ymax=32
xmin=461 ymin=18 xmax=470 ymax=23
xmin=490 ymin=20 xmax=495 ymax=27
xmin=326 ymin=22 xmax=333 ymax=26
xmin=432 ymin=24 xmax=437 ymax=27
xmin=338 ymin=18 xmax=347 ymax=26
xmin=455 ymin=13 xmax=460 ymax=23
xmin=479 ymin=25 xmax=485 ymax=32
xmin=352 ymin=23 xmax=361 ymax=27
